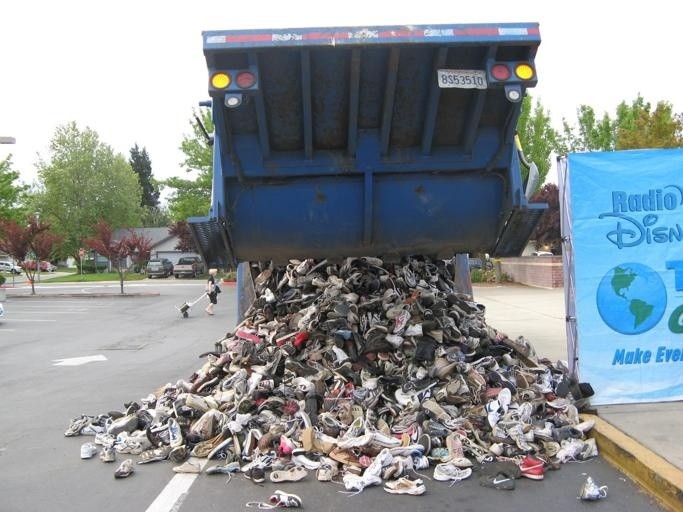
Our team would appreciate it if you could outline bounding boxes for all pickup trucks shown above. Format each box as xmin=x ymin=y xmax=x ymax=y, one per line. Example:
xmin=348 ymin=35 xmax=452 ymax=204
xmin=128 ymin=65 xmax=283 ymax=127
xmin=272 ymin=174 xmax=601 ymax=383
xmin=173 ymin=258 xmax=205 ymax=277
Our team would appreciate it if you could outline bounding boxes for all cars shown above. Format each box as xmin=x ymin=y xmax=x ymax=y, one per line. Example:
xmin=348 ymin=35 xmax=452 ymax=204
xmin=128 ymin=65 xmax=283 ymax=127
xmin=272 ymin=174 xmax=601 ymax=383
xmin=528 ymin=250 xmax=553 ymax=257
xmin=0 ymin=303 xmax=4 ymax=319
xmin=0 ymin=261 xmax=57 ymax=274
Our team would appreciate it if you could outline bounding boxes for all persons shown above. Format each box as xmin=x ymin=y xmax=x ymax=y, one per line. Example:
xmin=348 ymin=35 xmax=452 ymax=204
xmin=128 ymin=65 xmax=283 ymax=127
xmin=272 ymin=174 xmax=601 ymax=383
xmin=205 ymin=267 xmax=220 ymax=316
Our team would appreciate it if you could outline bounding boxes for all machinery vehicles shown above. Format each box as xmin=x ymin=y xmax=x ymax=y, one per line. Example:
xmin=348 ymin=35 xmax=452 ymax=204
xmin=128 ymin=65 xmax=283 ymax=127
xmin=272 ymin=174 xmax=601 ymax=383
xmin=181 ymin=20 xmax=550 ymax=326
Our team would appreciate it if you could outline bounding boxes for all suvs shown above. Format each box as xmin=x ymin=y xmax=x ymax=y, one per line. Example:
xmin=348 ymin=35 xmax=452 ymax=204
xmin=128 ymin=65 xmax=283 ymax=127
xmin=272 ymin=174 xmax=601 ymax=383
xmin=145 ymin=259 xmax=173 ymax=278
xmin=468 ymin=258 xmax=491 ymax=272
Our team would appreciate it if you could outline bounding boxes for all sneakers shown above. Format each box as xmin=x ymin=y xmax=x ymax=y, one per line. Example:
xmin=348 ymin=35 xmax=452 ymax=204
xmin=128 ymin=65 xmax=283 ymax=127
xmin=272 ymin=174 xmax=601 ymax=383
xmin=270 ymin=489 xmax=305 ymax=507
xmin=578 ymin=477 xmax=611 ymax=503
